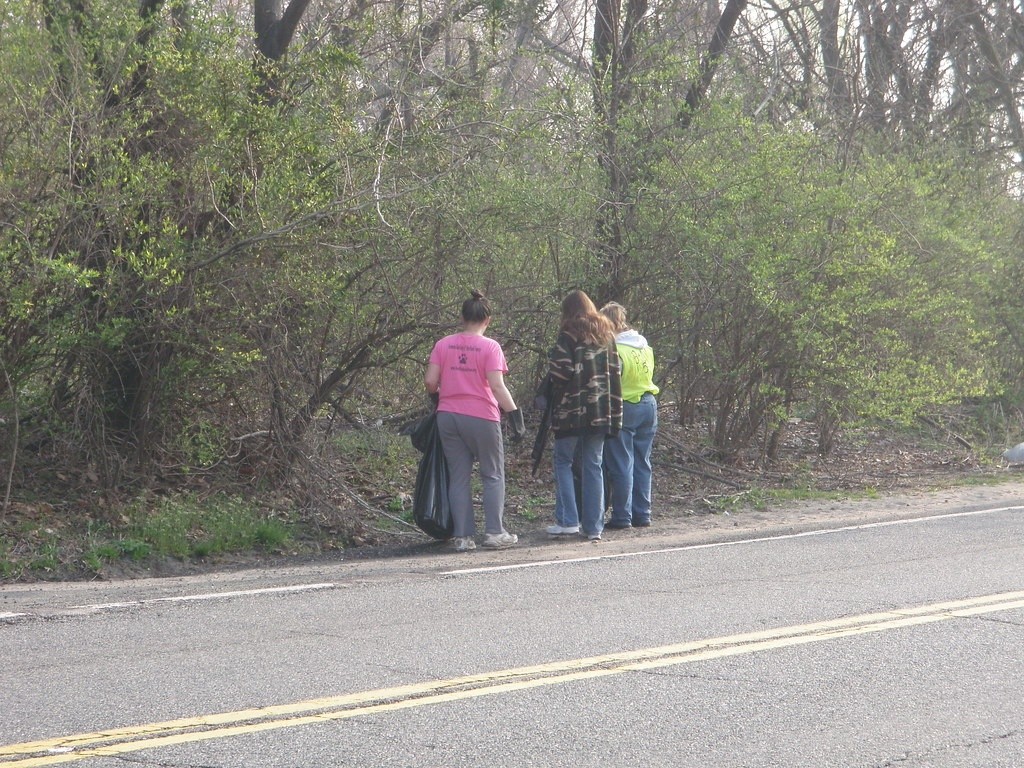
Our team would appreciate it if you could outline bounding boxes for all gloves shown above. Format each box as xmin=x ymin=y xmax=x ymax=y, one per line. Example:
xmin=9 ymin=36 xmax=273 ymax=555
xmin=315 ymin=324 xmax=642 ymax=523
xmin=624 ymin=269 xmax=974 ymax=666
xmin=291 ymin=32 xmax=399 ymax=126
xmin=506 ymin=407 xmax=527 ymax=446
xmin=428 ymin=387 xmax=439 ymax=406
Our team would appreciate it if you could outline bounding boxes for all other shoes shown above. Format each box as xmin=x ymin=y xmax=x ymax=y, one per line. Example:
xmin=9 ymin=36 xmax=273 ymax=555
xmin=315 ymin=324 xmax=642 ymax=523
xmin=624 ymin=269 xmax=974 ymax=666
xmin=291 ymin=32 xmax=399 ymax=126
xmin=632 ymin=519 xmax=651 ymax=526
xmin=608 ymin=520 xmax=632 ymax=529
xmin=603 ymin=506 xmax=614 ymax=525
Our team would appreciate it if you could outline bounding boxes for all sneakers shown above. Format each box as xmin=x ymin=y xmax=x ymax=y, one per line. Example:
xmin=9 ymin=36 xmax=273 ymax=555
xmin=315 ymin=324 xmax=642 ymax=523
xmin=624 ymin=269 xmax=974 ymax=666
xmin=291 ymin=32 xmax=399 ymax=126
xmin=454 ymin=537 xmax=477 ymax=552
xmin=586 ymin=534 xmax=602 ymax=540
xmin=480 ymin=532 xmax=519 ymax=550
xmin=545 ymin=524 xmax=580 ymax=535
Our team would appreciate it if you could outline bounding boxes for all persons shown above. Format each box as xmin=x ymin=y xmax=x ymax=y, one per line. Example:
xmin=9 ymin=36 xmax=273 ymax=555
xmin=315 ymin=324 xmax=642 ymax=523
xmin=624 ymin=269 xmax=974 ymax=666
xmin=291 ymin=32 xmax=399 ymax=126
xmin=424 ymin=288 xmax=525 ymax=550
xmin=598 ymin=301 xmax=660 ymax=528
xmin=546 ymin=290 xmax=622 ymax=539
